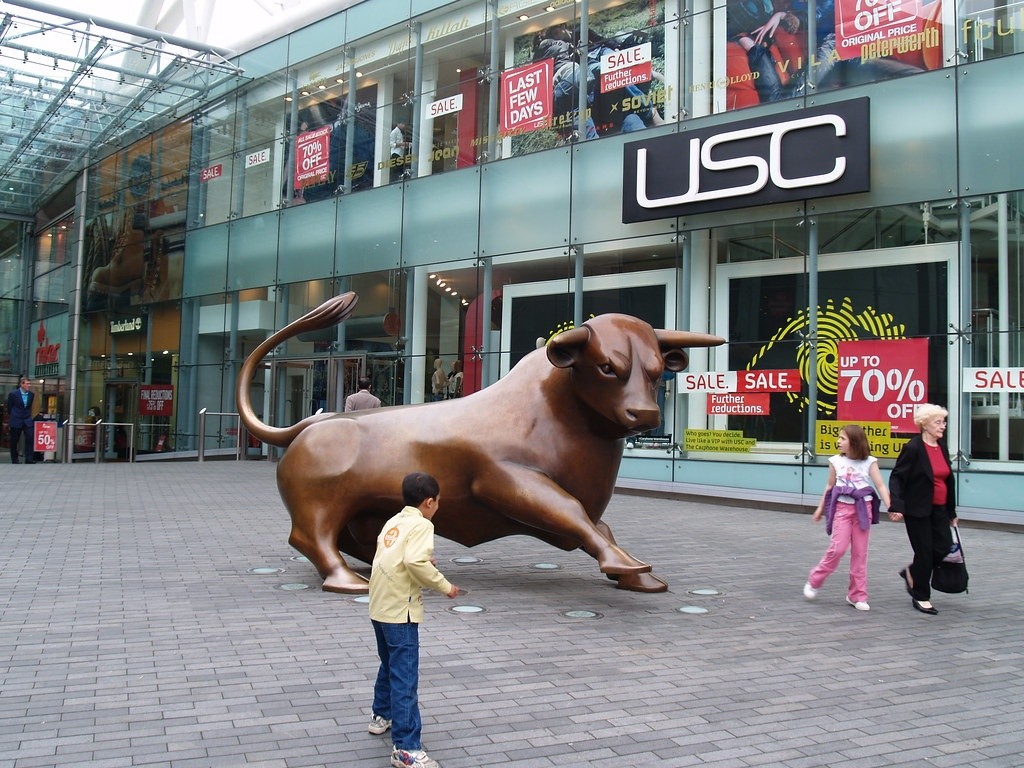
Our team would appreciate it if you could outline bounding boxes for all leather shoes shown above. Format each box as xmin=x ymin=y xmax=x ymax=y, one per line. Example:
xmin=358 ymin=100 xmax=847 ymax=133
xmin=913 ymin=598 xmax=938 ymax=614
xmin=899 ymin=568 xmax=917 ymax=600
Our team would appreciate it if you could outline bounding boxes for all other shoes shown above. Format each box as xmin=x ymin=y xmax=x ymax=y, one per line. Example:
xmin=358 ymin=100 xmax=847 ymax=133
xmin=846 ymin=595 xmax=871 ymax=611
xmin=802 ymin=582 xmax=818 ymax=599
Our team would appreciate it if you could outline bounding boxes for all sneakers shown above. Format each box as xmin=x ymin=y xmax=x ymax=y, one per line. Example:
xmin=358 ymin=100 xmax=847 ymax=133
xmin=367 ymin=712 xmax=393 ymax=735
xmin=390 ymin=743 xmax=442 ymax=768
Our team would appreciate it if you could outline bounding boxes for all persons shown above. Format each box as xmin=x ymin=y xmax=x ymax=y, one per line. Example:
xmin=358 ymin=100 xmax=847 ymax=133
xmin=389 ymin=119 xmax=409 ymax=184
xmin=802 ymin=425 xmax=893 ymax=612
xmin=365 ymin=472 xmax=461 ymax=768
xmin=6 ymin=377 xmax=36 ymax=464
xmin=345 ymin=377 xmax=381 ymax=413
xmin=447 ymin=362 xmax=455 ymax=379
xmin=450 ymin=361 xmax=463 ymax=398
xmin=887 ymin=404 xmax=959 ymax=615
xmin=534 ymin=22 xmax=667 ymax=142
xmin=432 ymin=359 xmax=450 ymax=402
xmin=726 ymin=0 xmax=928 ymax=104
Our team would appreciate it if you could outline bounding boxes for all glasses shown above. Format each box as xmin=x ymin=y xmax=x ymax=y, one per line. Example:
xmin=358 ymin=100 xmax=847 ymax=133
xmin=930 ymin=421 xmax=946 ymax=426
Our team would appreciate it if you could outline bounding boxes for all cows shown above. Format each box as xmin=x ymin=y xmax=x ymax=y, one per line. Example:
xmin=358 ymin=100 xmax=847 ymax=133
xmin=235 ymin=291 xmax=726 ymax=594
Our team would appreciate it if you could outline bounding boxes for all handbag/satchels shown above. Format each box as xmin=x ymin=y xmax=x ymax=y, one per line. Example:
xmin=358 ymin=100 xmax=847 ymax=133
xmin=932 ymin=521 xmax=970 ymax=594
